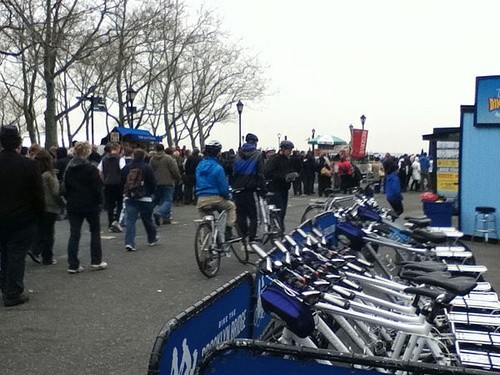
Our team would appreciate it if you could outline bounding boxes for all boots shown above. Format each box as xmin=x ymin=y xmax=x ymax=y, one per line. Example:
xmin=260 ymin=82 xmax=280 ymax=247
xmin=223 ymin=225 xmax=241 ymax=243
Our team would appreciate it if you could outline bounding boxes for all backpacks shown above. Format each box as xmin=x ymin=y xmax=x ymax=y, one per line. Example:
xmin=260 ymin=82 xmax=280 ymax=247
xmin=123 ymin=161 xmax=148 ymax=199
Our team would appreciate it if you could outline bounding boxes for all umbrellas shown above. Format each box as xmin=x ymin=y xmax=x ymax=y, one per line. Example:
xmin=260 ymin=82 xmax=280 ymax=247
xmin=307 ymin=134 xmax=345 ymax=150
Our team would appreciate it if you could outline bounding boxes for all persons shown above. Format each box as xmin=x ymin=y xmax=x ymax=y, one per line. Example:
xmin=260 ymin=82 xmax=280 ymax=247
xmin=195 ymin=141 xmax=237 ymax=268
xmin=63 ymin=141 xmax=107 ymax=273
xmin=19 ymin=142 xmax=78 ymax=265
xmin=149 ymin=145 xmax=180 ymax=226
xmin=264 ymin=141 xmax=296 ymax=238
xmin=218 ymin=148 xmax=435 ymax=192
xmin=165 ymin=146 xmax=204 ymax=206
xmin=86 ymin=141 xmax=156 ymax=233
xmin=231 ymin=134 xmax=267 ymax=243
xmin=385 ymin=165 xmax=404 ymax=221
xmin=119 ymin=148 xmax=161 ymax=250
xmin=0 ymin=125 xmax=45 ymax=306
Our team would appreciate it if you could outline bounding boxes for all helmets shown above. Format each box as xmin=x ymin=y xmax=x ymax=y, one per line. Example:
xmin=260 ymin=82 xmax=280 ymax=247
xmin=279 ymin=140 xmax=294 ymax=149
xmin=245 ymin=133 xmax=259 ymax=142
xmin=204 ymin=140 xmax=222 ymax=152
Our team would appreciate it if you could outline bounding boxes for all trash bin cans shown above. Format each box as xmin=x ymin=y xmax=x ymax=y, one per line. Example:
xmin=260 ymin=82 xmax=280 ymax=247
xmin=423 ymin=200 xmax=455 ymax=227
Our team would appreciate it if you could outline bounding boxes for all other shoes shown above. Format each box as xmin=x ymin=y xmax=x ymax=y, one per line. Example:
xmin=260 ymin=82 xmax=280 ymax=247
xmin=67 ymin=265 xmax=84 ymax=274
xmin=125 ymin=244 xmax=137 ymax=252
xmin=108 ymin=220 xmax=123 ymax=233
xmin=27 ymin=250 xmax=41 ymax=264
xmin=148 ymin=235 xmax=161 ymax=246
xmin=162 ymin=220 xmax=172 ymax=225
xmin=91 ymin=261 xmax=108 ymax=268
xmin=153 ymin=213 xmax=161 ymax=226
xmin=42 ymin=259 xmax=58 ymax=265
xmin=1 ymin=290 xmax=31 ymax=307
xmin=248 ymin=237 xmax=263 ymax=244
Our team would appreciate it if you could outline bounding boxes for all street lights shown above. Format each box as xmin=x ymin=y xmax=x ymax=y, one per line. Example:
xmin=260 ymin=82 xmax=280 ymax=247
xmin=349 ymin=123 xmax=353 ymax=142
xmin=311 ymin=128 xmax=315 ymax=156
xmin=125 ymin=87 xmax=137 ymax=128
xmin=360 ymin=114 xmax=366 ymax=130
xmin=236 ymin=99 xmax=244 ymax=148
xmin=84 ymin=85 xmax=99 ymax=145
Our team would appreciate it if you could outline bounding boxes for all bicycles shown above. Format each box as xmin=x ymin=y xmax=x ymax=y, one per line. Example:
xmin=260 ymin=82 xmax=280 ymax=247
xmin=195 ymin=186 xmax=500 ymax=375
xmin=192 ymin=187 xmax=249 ymax=279
xmin=253 ymin=179 xmax=284 ymax=247
